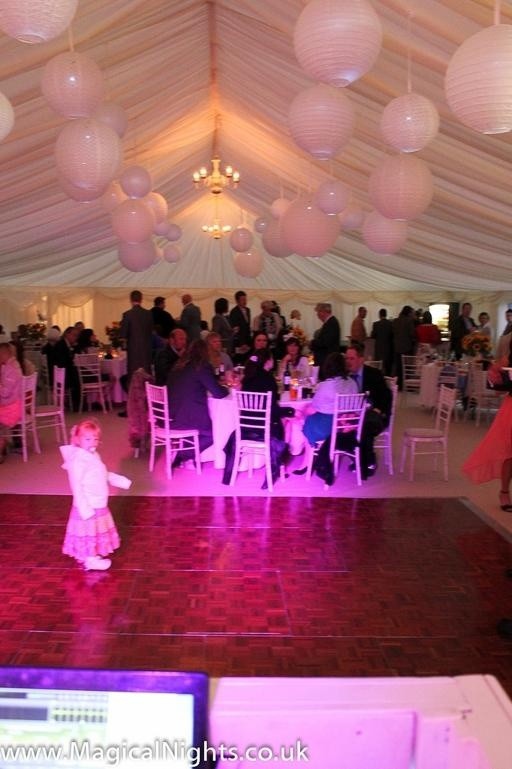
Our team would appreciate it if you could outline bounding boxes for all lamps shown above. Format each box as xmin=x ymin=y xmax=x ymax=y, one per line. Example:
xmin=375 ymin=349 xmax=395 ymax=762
xmin=200 ymin=218 xmax=236 ymax=242
xmin=190 ymin=155 xmax=242 ymax=196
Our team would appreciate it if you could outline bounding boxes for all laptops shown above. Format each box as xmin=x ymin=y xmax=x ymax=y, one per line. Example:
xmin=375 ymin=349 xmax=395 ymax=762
xmin=0 ymin=666 xmax=208 ymax=769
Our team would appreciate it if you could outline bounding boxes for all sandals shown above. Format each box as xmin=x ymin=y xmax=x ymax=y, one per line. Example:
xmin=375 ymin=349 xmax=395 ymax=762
xmin=499 ymin=489 xmax=512 ymax=512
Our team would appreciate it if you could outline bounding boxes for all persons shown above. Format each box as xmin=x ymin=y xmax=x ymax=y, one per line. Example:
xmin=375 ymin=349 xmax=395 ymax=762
xmin=59 ymin=419 xmax=133 ymax=570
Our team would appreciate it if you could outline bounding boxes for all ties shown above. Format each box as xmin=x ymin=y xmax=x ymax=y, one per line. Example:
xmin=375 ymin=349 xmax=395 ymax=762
xmin=352 ymin=374 xmax=361 ymax=391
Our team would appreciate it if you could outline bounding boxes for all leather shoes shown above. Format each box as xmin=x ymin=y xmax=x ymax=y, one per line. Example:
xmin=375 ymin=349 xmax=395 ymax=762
xmin=118 ymin=411 xmax=128 ymax=418
xmin=292 ymin=464 xmax=308 ymax=477
xmin=347 ymin=461 xmax=378 ymax=476
xmin=171 ymin=452 xmax=183 ymax=469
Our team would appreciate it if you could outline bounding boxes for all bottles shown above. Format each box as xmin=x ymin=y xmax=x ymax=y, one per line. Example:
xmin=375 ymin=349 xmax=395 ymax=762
xmin=288 ymin=372 xmax=298 ymax=401
xmin=214 ymin=366 xmax=220 ymax=380
xmin=282 ymin=362 xmax=292 ymax=390
xmin=219 ymin=361 xmax=225 ymax=380
xmin=296 ymin=375 xmax=319 ymax=399
xmin=440 ymin=348 xmax=456 ymax=362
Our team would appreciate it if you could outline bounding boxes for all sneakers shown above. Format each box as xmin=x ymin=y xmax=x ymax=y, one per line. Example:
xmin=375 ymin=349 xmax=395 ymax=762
xmin=77 ymin=555 xmax=112 ymax=572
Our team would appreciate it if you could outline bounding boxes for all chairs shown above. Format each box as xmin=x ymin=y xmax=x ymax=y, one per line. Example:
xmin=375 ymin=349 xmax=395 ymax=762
xmin=0 ymin=320 xmax=510 ymax=495
